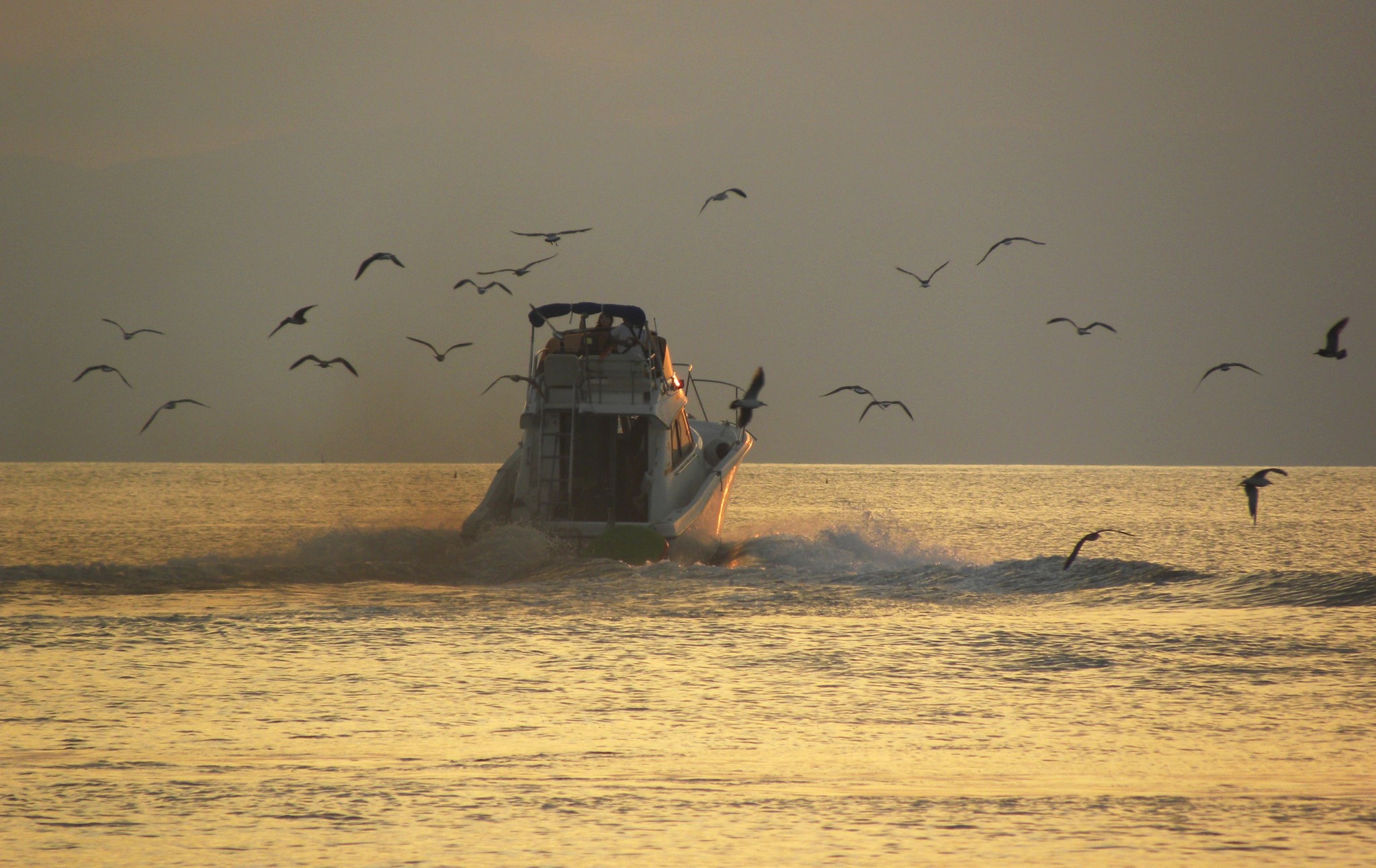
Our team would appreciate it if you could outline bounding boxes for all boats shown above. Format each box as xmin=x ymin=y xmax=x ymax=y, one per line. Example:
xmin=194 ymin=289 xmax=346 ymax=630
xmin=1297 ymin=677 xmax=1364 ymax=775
xmin=455 ymin=298 xmax=756 ymax=567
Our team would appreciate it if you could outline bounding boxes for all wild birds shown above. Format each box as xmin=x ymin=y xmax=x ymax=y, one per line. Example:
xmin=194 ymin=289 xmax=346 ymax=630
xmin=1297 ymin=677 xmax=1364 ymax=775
xmin=1062 ymin=529 xmax=1135 ymax=571
xmin=1313 ymin=317 xmax=1349 ymax=360
xmin=77 ymin=188 xmax=765 ymax=438
xmin=976 ymin=237 xmax=1046 ymax=266
xmin=896 ymin=260 xmax=950 ymax=288
xmin=1048 ymin=318 xmax=1118 ymax=335
xmin=1238 ymin=468 xmax=1288 ymax=525
xmin=820 ymin=385 xmax=915 ymax=423
xmin=1194 ymin=363 xmax=1263 ymax=387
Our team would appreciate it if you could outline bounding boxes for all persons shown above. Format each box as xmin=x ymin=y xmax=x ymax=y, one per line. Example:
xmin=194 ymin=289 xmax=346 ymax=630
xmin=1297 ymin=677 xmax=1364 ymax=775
xmin=610 ymin=319 xmax=646 ymax=357
